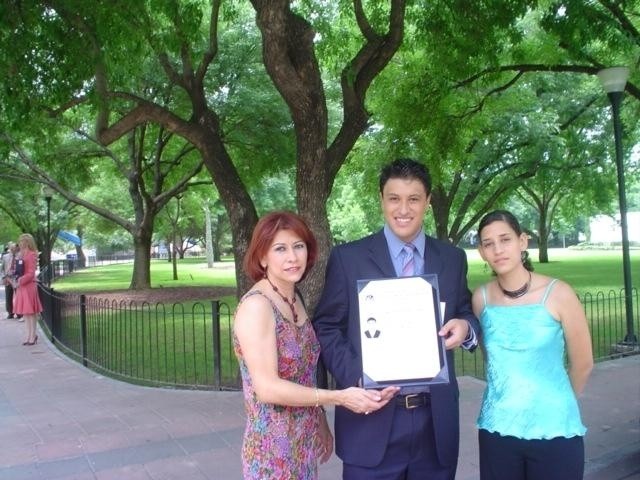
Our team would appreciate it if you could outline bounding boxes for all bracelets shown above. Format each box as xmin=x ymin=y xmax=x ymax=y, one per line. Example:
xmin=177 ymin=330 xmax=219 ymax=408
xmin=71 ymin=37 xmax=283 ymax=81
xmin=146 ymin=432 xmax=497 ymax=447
xmin=315 ymin=390 xmax=320 ymax=408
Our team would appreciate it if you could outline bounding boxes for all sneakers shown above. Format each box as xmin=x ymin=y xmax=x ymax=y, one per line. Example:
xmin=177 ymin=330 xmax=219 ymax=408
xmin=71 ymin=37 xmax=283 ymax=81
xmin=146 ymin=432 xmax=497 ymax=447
xmin=7 ymin=313 xmax=23 ymax=319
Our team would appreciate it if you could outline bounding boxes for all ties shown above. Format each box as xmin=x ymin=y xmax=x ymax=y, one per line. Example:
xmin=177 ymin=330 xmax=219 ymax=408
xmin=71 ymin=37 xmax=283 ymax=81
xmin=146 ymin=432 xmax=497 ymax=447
xmin=10 ymin=254 xmax=16 ymax=274
xmin=401 ymin=241 xmax=417 ymax=278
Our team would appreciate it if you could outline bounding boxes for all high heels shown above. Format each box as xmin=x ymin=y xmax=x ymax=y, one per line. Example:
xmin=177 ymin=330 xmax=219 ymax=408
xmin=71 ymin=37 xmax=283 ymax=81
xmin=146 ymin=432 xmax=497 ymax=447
xmin=22 ymin=335 xmax=38 ymax=345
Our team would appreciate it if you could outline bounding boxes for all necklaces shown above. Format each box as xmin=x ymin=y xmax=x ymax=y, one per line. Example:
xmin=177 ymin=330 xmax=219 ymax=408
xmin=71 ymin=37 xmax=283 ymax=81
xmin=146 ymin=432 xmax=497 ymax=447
xmin=495 ymin=270 xmax=533 ymax=299
xmin=263 ymin=273 xmax=298 ymax=323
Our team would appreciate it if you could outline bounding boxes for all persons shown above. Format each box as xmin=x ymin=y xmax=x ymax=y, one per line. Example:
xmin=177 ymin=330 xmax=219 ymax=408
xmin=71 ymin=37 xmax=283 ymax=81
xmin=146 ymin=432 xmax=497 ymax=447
xmin=364 ymin=318 xmax=381 ymax=339
xmin=228 ymin=209 xmax=391 ymax=479
xmin=1 ymin=233 xmax=43 ymax=346
xmin=0 ymin=242 xmax=26 ymax=322
xmin=470 ymin=209 xmax=596 ymax=480
xmin=309 ymin=157 xmax=484 ymax=479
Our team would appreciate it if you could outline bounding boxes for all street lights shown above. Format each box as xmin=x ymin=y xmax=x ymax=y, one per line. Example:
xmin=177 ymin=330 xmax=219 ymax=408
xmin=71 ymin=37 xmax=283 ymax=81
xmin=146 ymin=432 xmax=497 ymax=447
xmin=598 ymin=66 xmax=638 ymax=355
xmin=42 ymin=187 xmax=55 ymax=288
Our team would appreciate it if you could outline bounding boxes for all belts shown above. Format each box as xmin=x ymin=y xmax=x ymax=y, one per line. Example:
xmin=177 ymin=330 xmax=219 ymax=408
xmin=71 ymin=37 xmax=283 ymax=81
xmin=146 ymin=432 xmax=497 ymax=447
xmin=395 ymin=392 xmax=432 ymax=410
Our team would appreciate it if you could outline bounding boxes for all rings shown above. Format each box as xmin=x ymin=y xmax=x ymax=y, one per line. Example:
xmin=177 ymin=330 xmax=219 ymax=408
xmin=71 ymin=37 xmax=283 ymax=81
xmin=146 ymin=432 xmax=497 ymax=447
xmin=365 ymin=411 xmax=371 ymax=416
